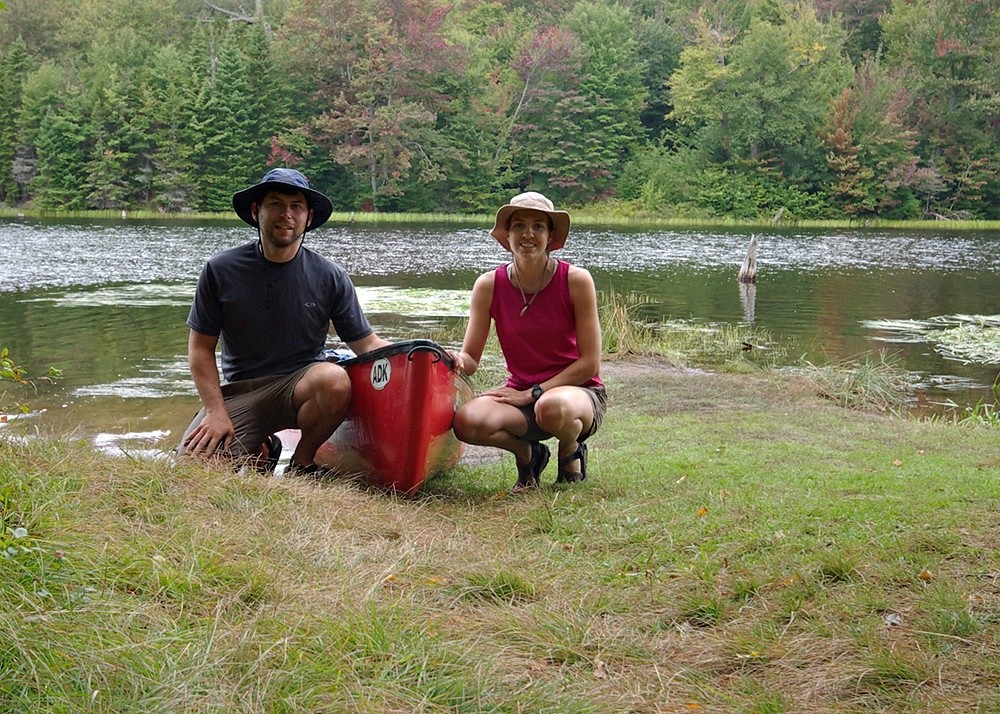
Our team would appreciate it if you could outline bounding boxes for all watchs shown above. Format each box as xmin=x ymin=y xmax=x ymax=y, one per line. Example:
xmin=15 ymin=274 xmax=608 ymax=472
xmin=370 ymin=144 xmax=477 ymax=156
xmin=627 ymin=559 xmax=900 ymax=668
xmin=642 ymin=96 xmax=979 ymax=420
xmin=532 ymin=384 xmax=543 ymax=398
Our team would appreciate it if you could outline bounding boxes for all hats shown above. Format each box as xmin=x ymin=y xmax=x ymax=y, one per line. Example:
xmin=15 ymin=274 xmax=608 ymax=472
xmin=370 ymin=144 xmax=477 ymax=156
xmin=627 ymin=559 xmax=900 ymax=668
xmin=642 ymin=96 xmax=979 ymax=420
xmin=493 ymin=191 xmax=569 ymax=250
xmin=233 ymin=168 xmax=333 ymax=233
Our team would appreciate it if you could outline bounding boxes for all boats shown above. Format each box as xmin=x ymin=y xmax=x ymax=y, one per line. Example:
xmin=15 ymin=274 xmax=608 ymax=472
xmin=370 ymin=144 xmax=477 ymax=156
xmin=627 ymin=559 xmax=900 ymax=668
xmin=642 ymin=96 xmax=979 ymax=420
xmin=316 ymin=335 xmax=473 ymax=500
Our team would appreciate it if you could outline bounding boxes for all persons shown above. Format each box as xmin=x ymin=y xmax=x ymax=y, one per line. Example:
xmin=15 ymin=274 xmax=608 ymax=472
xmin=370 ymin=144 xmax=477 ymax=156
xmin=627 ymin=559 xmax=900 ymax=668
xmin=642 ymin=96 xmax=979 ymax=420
xmin=179 ymin=168 xmax=392 ymax=480
xmin=446 ymin=192 xmax=608 ymax=493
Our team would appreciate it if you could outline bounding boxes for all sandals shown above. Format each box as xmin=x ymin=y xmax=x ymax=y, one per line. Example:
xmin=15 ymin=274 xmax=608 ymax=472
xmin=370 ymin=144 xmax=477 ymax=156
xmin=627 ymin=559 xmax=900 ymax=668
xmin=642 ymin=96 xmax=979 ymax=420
xmin=511 ymin=441 xmax=551 ymax=494
xmin=234 ymin=434 xmax=281 ymax=480
xmin=283 ymin=463 xmax=341 ymax=480
xmin=553 ymin=443 xmax=588 ymax=489
